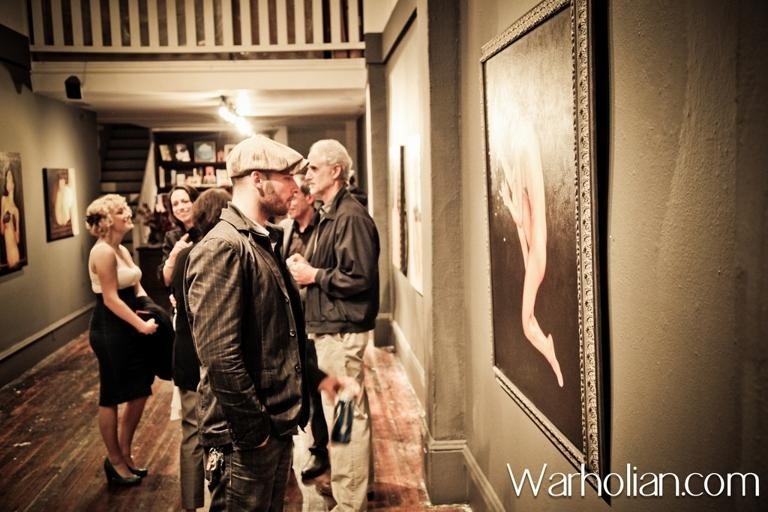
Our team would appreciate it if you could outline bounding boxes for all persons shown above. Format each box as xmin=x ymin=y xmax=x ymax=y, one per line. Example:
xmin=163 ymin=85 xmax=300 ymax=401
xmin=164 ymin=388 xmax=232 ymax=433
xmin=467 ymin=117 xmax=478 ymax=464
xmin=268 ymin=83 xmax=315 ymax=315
xmin=54 ymin=176 xmax=70 ymax=226
xmin=498 ymin=106 xmax=564 ymax=389
xmin=86 ymin=135 xmax=379 ymax=512
xmin=1 ymin=165 xmax=20 ymax=267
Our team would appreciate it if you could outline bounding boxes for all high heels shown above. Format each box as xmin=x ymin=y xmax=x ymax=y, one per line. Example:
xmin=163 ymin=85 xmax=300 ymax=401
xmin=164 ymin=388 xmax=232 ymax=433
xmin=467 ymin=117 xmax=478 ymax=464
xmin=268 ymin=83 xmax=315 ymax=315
xmin=104 ymin=456 xmax=147 ymax=486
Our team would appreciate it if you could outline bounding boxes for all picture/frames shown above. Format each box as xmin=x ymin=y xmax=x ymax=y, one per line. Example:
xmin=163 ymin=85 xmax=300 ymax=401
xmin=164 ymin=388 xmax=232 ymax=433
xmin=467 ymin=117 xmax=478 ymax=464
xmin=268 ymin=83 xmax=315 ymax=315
xmin=0 ymin=151 xmax=30 ymax=277
xmin=481 ymin=1 xmax=611 ymax=507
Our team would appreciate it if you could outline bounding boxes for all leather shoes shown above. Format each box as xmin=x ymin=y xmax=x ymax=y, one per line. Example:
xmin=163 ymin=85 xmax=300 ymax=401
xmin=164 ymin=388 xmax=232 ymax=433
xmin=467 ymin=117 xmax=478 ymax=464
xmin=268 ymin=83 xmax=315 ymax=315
xmin=301 ymin=452 xmax=329 ymax=478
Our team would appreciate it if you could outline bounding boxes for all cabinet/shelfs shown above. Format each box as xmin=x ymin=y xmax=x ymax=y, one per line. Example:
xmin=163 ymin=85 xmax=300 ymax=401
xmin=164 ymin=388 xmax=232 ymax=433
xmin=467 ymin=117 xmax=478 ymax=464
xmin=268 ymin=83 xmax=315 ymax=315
xmin=141 ymin=123 xmax=290 ymax=238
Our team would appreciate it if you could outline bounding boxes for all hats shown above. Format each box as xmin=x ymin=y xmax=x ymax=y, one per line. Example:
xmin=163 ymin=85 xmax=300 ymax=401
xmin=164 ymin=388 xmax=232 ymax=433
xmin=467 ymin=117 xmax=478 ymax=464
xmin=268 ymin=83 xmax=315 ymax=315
xmin=226 ymin=135 xmax=308 ymax=177
